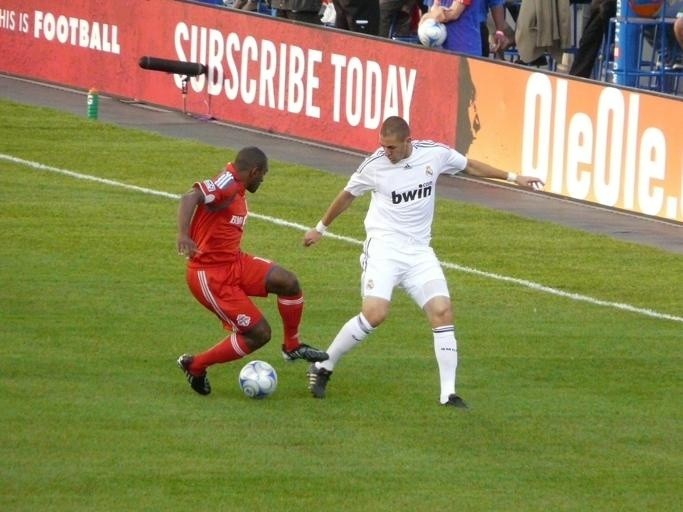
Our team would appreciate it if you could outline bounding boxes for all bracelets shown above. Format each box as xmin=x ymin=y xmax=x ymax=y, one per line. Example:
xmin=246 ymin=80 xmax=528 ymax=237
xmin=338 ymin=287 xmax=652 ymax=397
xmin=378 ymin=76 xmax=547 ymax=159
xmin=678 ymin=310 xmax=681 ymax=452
xmin=506 ymin=171 xmax=517 ymax=183
xmin=315 ymin=220 xmax=328 ymax=235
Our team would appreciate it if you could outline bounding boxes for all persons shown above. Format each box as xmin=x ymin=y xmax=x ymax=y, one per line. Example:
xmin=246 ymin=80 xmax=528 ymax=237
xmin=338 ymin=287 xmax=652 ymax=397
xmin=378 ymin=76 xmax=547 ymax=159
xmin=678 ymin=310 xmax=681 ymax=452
xmin=221 ymin=0 xmax=682 ymax=81
xmin=172 ymin=144 xmax=331 ymax=395
xmin=300 ymin=118 xmax=545 ymax=410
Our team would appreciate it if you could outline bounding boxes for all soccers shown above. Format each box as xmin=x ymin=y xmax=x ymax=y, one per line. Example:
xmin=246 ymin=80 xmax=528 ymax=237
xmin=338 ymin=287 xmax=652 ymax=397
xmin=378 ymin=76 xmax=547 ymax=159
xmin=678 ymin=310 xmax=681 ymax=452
xmin=417 ymin=18 xmax=447 ymax=47
xmin=240 ymin=359 xmax=277 ymax=399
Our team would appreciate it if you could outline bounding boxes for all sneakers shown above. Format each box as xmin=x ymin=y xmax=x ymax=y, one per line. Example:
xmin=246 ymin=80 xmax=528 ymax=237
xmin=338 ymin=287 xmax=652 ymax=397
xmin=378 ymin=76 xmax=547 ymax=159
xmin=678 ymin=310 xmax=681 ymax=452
xmin=177 ymin=354 xmax=210 ymax=395
xmin=281 ymin=342 xmax=329 ymax=362
xmin=307 ymin=363 xmax=333 ymax=399
xmin=440 ymin=394 xmax=468 ymax=409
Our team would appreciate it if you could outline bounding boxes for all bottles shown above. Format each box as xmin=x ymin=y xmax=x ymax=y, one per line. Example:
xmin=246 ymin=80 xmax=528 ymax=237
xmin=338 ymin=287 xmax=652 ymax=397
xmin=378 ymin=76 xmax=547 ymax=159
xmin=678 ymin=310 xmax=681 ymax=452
xmin=87 ymin=86 xmax=98 ymax=120
